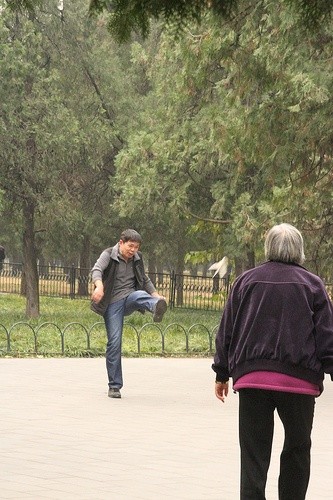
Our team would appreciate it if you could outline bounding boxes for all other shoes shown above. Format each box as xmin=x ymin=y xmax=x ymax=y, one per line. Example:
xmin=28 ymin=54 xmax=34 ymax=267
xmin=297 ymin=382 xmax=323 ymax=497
xmin=153 ymin=298 xmax=168 ymax=323
xmin=108 ymin=387 xmax=122 ymax=398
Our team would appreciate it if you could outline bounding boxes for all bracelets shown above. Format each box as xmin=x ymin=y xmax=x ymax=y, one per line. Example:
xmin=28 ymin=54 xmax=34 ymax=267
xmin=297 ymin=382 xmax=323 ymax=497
xmin=214 ymin=380 xmax=230 ymax=385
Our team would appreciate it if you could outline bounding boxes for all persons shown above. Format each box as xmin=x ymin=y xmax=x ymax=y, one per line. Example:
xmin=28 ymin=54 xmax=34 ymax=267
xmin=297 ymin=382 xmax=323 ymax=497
xmin=86 ymin=228 xmax=168 ymax=399
xmin=210 ymin=222 xmax=333 ymax=500
